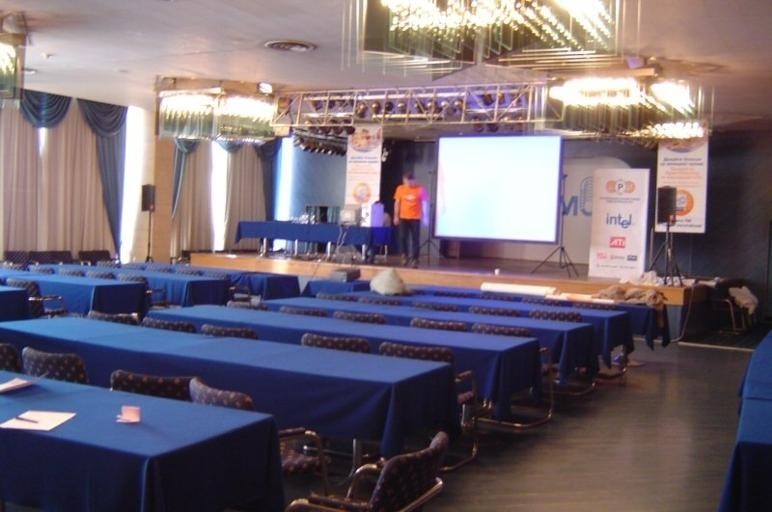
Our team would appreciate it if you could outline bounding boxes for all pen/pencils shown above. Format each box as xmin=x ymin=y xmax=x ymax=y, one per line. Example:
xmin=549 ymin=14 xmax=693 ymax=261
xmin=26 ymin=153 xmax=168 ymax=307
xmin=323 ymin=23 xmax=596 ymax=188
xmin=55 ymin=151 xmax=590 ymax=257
xmin=15 ymin=415 xmax=39 ymax=425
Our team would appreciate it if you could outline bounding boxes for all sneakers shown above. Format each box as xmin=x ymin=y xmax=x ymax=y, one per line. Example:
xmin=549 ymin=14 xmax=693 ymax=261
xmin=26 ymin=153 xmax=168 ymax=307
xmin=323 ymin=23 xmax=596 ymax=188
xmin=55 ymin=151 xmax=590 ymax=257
xmin=401 ymin=254 xmax=408 ymax=265
xmin=410 ymin=257 xmax=419 ymax=269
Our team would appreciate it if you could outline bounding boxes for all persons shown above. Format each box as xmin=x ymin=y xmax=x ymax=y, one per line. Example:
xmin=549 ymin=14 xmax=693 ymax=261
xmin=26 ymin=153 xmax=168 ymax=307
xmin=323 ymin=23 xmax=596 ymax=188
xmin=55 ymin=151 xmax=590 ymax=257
xmin=364 ymin=200 xmax=390 ymax=262
xmin=391 ymin=170 xmax=430 ymax=270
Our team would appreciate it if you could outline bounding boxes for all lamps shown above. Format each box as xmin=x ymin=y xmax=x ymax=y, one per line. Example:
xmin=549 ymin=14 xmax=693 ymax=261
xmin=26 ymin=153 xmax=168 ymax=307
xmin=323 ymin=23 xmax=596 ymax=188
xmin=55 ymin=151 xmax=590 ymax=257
xmin=365 ymin=1 xmax=624 ymax=69
xmin=290 ymin=83 xmax=541 ymax=160
xmin=0 ymin=12 xmax=33 ymax=111
xmin=554 ymin=75 xmax=714 ymax=142
xmin=154 ymin=76 xmax=276 ymax=144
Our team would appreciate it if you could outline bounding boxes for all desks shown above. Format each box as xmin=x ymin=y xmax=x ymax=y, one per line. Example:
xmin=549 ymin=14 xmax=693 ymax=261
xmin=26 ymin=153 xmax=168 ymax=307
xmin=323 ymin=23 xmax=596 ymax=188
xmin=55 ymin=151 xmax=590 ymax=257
xmin=720 ymin=329 xmax=771 ymax=509
xmin=234 ymin=221 xmax=393 ymax=265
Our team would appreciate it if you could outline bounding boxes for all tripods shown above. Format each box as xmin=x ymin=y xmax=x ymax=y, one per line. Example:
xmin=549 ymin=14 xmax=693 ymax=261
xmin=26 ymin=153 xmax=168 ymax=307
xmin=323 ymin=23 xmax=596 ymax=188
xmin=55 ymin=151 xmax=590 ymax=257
xmin=649 ymin=223 xmax=684 ymax=287
xmin=530 ymin=178 xmax=580 ymax=278
xmin=144 ymin=212 xmax=154 ymax=263
xmin=403 ymin=174 xmax=450 ymax=267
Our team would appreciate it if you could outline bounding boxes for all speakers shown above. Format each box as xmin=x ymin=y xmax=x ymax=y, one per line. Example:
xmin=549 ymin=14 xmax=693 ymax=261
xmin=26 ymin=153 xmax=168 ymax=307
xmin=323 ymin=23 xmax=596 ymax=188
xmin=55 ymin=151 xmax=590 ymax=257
xmin=305 ymin=205 xmax=327 ymax=254
xmin=142 ymin=185 xmax=155 ymax=212
xmin=658 ymin=186 xmax=676 ymax=223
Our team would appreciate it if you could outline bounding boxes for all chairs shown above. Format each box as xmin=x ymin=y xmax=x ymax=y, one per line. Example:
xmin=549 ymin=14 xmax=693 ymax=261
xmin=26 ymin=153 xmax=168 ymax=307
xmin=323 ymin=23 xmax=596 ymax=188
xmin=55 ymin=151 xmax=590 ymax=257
xmin=0 ymin=249 xmax=675 ymax=511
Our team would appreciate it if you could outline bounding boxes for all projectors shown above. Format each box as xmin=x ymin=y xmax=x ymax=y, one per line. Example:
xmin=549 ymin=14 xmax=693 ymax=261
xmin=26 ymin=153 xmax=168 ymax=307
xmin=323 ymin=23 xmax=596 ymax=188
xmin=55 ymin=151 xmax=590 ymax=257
xmin=330 ymin=267 xmax=360 ymax=282
